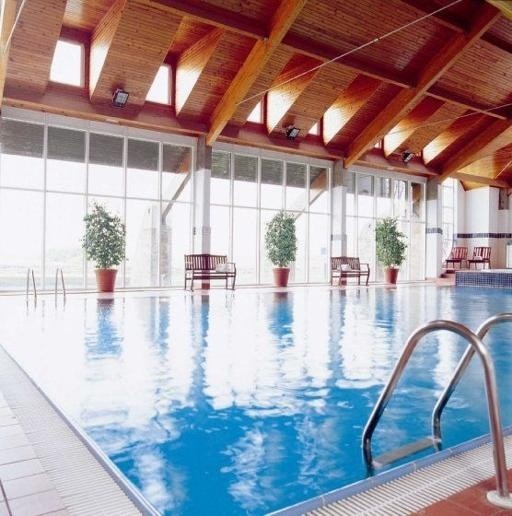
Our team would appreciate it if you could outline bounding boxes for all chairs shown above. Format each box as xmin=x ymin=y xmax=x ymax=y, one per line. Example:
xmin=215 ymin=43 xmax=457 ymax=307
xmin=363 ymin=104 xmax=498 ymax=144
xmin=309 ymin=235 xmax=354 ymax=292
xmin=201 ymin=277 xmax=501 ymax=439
xmin=446 ymin=247 xmax=467 ymax=270
xmin=468 ymin=247 xmax=492 ymax=270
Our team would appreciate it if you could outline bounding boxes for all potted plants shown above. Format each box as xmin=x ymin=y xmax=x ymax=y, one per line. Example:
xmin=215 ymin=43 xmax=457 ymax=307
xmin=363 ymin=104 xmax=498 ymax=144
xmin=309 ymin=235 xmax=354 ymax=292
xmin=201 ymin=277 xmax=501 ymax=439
xmin=374 ymin=218 xmax=408 ymax=284
xmin=80 ymin=198 xmax=129 ymax=292
xmin=264 ymin=210 xmax=298 ymax=286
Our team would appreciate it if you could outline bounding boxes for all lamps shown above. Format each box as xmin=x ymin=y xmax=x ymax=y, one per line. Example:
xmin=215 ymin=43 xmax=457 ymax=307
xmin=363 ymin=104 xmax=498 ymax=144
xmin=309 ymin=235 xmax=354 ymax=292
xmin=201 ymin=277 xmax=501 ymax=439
xmin=111 ymin=90 xmax=129 ymax=107
xmin=287 ymin=128 xmax=300 ymax=139
xmin=403 ymin=151 xmax=415 ymax=164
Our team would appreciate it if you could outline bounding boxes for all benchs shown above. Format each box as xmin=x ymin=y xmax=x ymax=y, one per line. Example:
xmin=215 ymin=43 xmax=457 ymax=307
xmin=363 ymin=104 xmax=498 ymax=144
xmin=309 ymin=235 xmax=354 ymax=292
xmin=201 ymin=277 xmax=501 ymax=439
xmin=330 ymin=256 xmax=370 ymax=285
xmin=184 ymin=254 xmax=236 ymax=290
